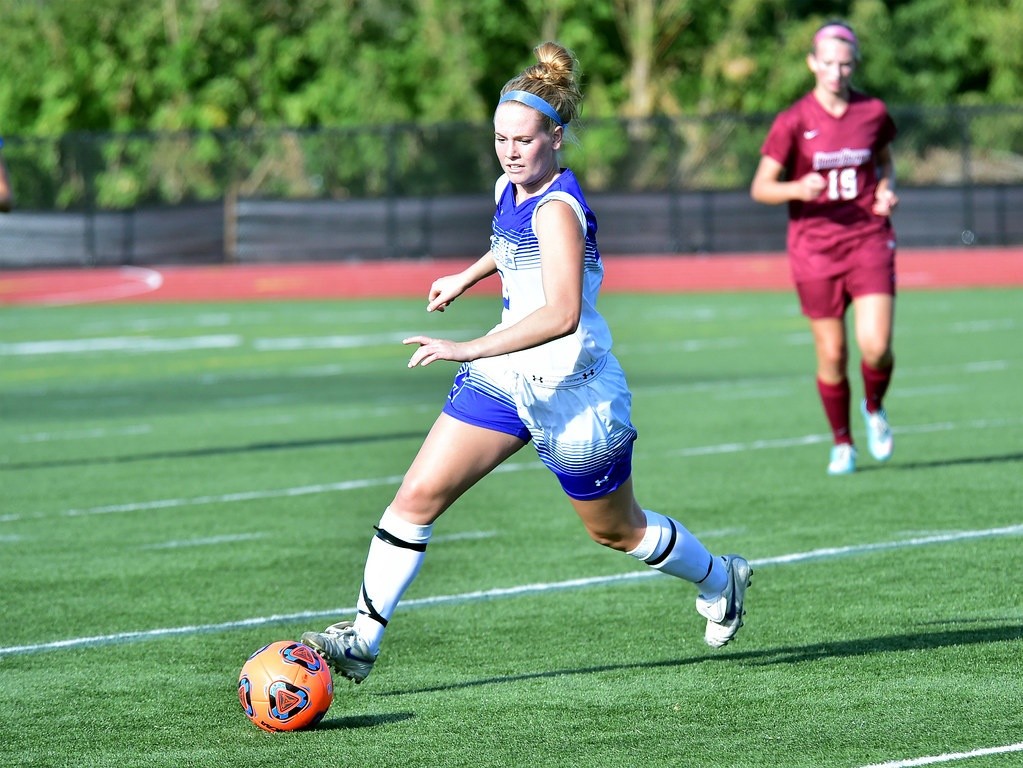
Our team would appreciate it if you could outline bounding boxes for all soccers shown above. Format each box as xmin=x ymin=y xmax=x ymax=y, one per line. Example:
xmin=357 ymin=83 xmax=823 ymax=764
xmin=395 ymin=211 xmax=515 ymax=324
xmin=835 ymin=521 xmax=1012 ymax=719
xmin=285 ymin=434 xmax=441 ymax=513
xmin=237 ymin=641 xmax=333 ymax=732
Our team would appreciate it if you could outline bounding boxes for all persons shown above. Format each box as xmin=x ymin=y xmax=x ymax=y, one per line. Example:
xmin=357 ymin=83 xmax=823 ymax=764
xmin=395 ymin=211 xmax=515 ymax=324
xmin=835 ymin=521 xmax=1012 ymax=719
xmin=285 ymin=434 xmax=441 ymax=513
xmin=746 ymin=21 xmax=899 ymax=475
xmin=301 ymin=41 xmax=756 ymax=685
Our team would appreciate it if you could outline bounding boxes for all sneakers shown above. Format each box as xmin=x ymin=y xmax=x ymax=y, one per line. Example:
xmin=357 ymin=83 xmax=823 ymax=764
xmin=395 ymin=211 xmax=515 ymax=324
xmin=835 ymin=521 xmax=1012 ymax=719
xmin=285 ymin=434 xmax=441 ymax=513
xmin=862 ymin=399 xmax=894 ymax=461
xmin=301 ymin=620 xmax=380 ymax=685
xmin=826 ymin=444 xmax=857 ymax=475
xmin=695 ymin=554 xmax=754 ymax=648
